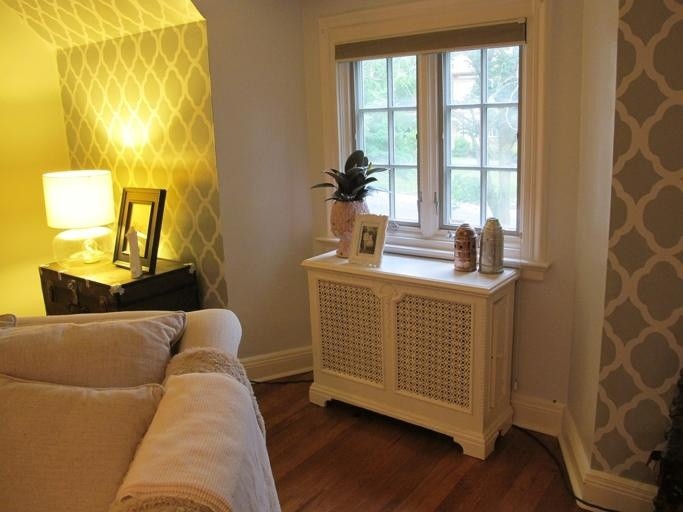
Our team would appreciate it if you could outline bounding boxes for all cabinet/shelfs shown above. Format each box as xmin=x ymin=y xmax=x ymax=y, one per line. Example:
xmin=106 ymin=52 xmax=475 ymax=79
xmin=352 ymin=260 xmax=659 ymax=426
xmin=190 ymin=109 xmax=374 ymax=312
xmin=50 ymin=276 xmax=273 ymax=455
xmin=300 ymin=248 xmax=522 ymax=461
xmin=37 ymin=254 xmax=202 ymax=316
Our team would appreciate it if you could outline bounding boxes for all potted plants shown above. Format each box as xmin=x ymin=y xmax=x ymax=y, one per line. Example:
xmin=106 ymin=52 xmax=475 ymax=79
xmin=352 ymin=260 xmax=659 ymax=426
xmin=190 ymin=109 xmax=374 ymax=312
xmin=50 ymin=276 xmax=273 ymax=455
xmin=308 ymin=149 xmax=391 ymax=258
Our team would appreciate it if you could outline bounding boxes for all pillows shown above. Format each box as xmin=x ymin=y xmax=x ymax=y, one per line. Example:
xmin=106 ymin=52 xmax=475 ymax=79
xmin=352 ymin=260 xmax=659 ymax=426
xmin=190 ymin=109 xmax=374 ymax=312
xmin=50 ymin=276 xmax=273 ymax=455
xmin=1 ymin=310 xmax=189 ymax=512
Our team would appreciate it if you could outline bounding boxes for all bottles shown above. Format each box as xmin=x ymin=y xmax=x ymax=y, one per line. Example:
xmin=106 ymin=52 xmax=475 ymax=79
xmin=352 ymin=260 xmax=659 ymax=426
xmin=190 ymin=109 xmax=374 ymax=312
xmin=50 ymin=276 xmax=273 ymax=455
xmin=126 ymin=226 xmax=143 ymax=279
xmin=453 ymin=216 xmax=504 ymax=275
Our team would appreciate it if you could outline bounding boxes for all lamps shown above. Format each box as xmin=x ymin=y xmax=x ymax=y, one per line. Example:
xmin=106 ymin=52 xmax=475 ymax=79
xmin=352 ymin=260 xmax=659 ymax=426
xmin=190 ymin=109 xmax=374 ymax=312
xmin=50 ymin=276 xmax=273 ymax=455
xmin=42 ymin=169 xmax=117 ymax=264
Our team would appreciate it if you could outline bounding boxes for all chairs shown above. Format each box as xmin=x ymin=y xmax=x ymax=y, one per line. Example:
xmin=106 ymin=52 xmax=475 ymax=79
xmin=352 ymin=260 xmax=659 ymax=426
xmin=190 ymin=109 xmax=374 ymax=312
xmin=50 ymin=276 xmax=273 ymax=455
xmin=1 ymin=307 xmax=283 ymax=512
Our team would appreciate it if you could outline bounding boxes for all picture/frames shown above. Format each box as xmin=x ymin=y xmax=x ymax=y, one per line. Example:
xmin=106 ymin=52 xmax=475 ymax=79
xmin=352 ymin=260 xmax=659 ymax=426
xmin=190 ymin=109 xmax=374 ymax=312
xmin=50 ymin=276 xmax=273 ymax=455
xmin=347 ymin=212 xmax=390 ymax=269
xmin=112 ymin=186 xmax=167 ymax=275
